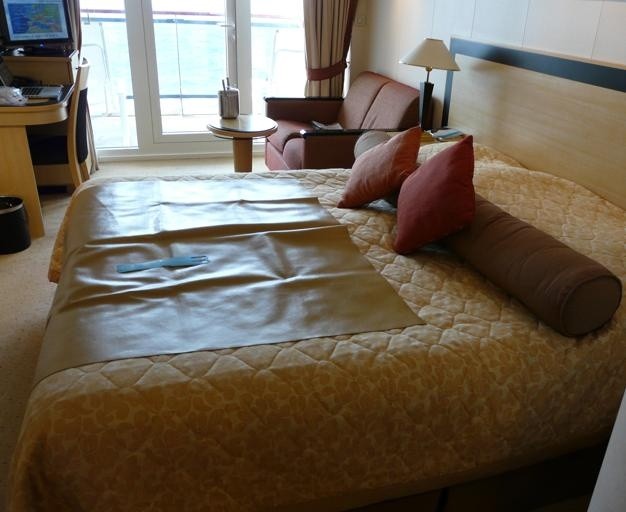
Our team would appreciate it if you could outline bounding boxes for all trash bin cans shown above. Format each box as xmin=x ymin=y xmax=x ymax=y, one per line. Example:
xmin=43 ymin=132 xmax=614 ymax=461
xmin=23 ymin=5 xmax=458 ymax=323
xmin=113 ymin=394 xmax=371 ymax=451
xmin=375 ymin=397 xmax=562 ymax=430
xmin=0 ymin=195 xmax=32 ymax=255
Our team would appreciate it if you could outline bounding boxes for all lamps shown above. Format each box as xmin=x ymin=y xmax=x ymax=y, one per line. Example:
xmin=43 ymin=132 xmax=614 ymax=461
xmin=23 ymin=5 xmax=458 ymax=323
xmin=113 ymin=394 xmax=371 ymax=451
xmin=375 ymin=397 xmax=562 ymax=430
xmin=396 ymin=39 xmax=463 ymax=131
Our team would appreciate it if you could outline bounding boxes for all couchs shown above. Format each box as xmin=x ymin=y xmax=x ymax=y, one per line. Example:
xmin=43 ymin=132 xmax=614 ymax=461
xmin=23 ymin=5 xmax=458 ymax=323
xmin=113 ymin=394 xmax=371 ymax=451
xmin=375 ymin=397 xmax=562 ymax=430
xmin=262 ymin=69 xmax=421 ymax=170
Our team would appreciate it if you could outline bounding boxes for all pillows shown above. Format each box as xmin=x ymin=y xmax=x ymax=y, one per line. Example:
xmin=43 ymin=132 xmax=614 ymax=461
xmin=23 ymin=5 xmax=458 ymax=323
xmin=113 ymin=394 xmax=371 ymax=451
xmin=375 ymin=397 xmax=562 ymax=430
xmin=393 ymin=135 xmax=476 ymax=251
xmin=339 ymin=125 xmax=425 ymax=206
xmin=435 ymin=194 xmax=621 ymax=335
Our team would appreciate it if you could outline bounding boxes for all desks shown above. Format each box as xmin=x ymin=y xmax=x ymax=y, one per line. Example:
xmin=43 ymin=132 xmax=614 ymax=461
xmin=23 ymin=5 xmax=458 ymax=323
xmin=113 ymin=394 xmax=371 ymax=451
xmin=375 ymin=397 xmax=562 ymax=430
xmin=206 ymin=114 xmax=279 ymax=172
xmin=0 ymin=49 xmax=79 ymax=239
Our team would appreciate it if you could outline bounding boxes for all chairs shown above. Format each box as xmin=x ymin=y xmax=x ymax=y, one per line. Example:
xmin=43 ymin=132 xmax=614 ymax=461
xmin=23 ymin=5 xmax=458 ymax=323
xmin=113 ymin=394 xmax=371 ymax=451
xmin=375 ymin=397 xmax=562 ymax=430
xmin=34 ymin=56 xmax=96 ymax=202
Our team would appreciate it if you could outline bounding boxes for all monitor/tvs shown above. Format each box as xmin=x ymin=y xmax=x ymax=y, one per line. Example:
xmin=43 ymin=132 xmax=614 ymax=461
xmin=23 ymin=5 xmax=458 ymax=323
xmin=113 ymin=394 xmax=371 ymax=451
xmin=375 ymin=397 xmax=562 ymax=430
xmin=0 ymin=0 xmax=74 ymax=53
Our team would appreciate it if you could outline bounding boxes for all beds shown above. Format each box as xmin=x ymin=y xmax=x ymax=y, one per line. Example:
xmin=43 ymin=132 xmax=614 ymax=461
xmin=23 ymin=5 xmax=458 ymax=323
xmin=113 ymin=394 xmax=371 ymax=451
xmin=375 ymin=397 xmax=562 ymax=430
xmin=1 ymin=36 xmax=626 ymax=511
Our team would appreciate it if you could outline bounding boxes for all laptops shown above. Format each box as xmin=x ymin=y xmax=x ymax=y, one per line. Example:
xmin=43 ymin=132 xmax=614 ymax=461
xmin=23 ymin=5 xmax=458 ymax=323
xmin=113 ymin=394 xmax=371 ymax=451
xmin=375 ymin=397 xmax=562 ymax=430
xmin=0 ymin=62 xmax=62 ymax=98
